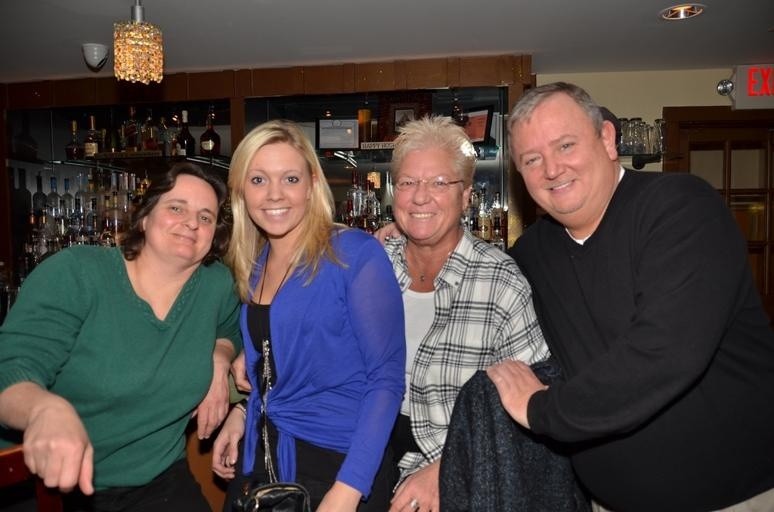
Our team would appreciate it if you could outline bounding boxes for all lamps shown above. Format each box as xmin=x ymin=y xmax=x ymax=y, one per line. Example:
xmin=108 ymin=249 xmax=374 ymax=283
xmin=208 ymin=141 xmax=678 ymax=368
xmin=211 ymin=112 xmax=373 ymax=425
xmin=112 ymin=0 xmax=165 ymax=87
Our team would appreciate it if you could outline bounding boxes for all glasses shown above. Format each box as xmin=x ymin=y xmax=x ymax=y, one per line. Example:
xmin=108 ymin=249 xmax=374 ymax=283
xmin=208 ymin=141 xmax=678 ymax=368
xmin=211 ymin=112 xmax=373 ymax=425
xmin=392 ymin=177 xmax=465 ymax=193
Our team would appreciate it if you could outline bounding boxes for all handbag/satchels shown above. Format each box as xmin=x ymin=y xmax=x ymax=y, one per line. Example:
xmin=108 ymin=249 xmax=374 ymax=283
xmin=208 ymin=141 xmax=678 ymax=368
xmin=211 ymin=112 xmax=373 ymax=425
xmin=244 ymin=482 xmax=312 ymax=511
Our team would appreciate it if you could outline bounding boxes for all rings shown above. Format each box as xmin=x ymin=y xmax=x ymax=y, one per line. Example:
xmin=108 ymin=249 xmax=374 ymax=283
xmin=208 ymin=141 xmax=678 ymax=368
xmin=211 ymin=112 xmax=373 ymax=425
xmin=409 ymin=499 xmax=421 ymax=512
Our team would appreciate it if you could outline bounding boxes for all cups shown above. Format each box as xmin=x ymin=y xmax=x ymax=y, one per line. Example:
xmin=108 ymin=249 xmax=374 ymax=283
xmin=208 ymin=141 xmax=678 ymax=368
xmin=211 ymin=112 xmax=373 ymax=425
xmin=619 ymin=118 xmax=666 ymax=155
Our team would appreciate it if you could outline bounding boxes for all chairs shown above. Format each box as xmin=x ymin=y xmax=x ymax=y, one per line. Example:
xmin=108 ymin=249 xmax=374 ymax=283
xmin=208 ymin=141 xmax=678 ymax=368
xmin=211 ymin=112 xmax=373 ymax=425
xmin=186 ymin=427 xmax=228 ymax=511
xmin=0 ymin=444 xmax=65 ymax=511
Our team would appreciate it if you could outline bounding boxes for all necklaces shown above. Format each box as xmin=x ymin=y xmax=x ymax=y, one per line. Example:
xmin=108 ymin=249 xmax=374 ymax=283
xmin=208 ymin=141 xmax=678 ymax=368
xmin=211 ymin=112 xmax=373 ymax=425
xmin=257 ymin=248 xmax=301 ymax=485
xmin=419 ymin=250 xmax=453 ymax=283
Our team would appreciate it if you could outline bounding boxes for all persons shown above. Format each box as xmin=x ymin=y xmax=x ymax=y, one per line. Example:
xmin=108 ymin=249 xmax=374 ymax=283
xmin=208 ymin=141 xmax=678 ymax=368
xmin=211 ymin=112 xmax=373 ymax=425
xmin=387 ymin=81 xmax=774 ymax=512
xmin=209 ymin=113 xmax=553 ymax=512
xmin=209 ymin=119 xmax=406 ymax=512
xmin=0 ymin=161 xmax=243 ymax=512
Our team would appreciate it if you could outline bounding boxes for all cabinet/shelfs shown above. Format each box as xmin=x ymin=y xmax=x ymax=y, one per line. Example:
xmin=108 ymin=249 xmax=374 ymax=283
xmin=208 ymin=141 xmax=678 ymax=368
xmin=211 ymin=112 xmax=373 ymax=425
xmin=1 ymin=144 xmax=234 ymax=339
xmin=661 ymin=106 xmax=773 ymax=332
xmin=1 ymin=52 xmax=536 ymax=245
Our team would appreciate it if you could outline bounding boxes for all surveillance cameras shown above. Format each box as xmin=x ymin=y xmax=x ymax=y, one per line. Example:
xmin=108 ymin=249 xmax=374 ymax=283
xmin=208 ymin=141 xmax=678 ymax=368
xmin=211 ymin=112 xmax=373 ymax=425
xmin=82 ymin=43 xmax=109 ymax=70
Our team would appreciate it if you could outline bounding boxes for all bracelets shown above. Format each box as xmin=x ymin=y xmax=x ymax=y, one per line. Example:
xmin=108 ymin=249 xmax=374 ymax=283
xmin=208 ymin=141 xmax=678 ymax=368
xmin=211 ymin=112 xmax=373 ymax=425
xmin=234 ymin=401 xmax=249 ymax=418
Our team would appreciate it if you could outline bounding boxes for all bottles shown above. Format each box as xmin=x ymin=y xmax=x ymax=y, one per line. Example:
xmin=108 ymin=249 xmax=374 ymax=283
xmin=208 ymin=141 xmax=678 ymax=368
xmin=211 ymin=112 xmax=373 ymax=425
xmin=0 ymin=165 xmax=152 ymax=318
xmin=64 ymin=104 xmax=220 ymax=158
xmin=339 ymin=168 xmax=507 ymax=241
